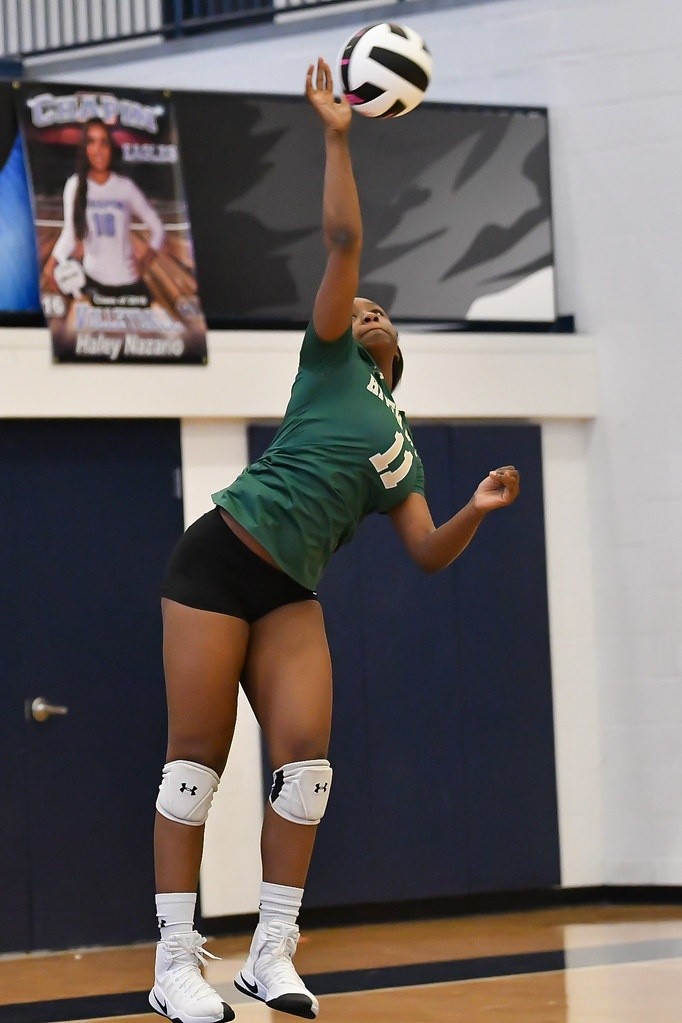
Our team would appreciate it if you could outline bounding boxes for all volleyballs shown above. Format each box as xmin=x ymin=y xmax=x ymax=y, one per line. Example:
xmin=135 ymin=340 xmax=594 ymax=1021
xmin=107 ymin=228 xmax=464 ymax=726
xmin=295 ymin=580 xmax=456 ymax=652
xmin=338 ymin=19 xmax=435 ymax=121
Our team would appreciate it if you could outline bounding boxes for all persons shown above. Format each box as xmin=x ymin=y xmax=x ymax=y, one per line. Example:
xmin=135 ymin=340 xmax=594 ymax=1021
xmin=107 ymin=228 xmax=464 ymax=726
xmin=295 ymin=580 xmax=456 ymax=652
xmin=43 ymin=118 xmax=165 ymax=332
xmin=146 ymin=59 xmax=522 ymax=1022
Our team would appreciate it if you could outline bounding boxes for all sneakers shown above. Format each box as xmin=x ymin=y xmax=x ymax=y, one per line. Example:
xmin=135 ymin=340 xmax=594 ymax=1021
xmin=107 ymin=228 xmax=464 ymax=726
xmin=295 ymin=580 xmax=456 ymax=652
xmin=234 ymin=919 xmax=319 ymax=1019
xmin=148 ymin=930 xmax=235 ymax=1023
